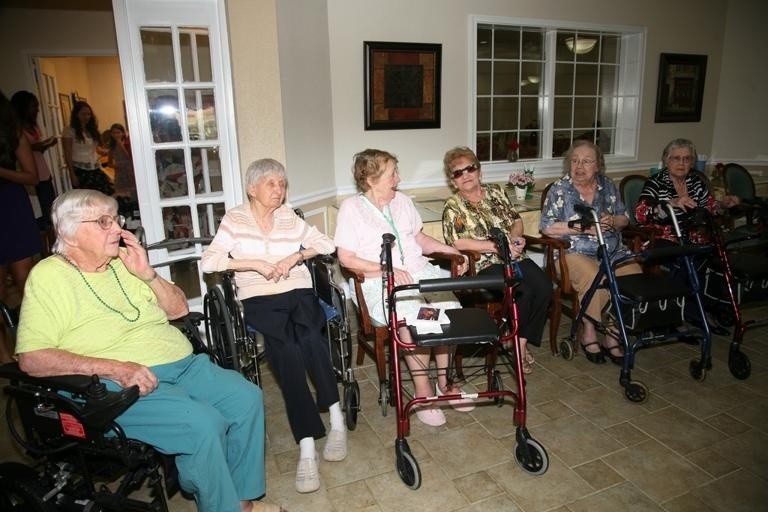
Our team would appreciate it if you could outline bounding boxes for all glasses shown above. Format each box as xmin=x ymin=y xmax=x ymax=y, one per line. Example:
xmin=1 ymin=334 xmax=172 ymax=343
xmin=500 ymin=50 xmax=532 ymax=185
xmin=670 ymin=155 xmax=692 ymax=163
xmin=571 ymin=159 xmax=596 ymax=166
xmin=451 ymin=164 xmax=478 ymax=179
xmin=81 ymin=215 xmax=126 ymax=230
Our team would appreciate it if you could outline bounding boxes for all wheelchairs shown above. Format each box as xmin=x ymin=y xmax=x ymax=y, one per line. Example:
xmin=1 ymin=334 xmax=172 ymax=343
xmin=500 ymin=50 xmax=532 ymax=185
xmin=3 ymin=300 xmax=204 ymax=512
xmin=185 ymin=208 xmax=362 ymax=430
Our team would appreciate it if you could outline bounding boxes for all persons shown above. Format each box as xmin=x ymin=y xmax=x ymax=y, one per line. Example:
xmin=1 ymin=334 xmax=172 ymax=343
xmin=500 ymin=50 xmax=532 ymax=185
xmin=10 ymin=90 xmax=58 ymax=257
xmin=633 ymin=138 xmax=731 ymax=345
xmin=539 ymin=141 xmax=630 ymax=368
xmin=0 ymin=88 xmax=40 ymax=305
xmin=334 ymin=148 xmax=475 ymax=426
xmin=61 ymin=101 xmax=114 ymax=195
xmin=12 ymin=189 xmax=288 ymax=512
xmin=107 ymin=123 xmax=135 ymax=196
xmin=441 ymin=147 xmax=552 ymax=375
xmin=201 ymin=158 xmax=347 ymax=493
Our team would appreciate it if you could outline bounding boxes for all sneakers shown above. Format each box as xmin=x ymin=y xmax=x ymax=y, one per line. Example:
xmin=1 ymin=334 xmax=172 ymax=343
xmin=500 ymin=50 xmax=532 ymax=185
xmin=412 ymin=393 xmax=447 ymax=427
xmin=295 ymin=452 xmax=320 ymax=493
xmin=322 ymin=428 xmax=348 ymax=462
xmin=435 ymin=381 xmax=476 ymax=412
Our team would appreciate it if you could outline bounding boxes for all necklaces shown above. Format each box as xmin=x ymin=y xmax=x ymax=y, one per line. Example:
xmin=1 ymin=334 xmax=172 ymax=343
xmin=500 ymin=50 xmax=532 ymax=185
xmin=54 ymin=250 xmax=140 ymax=322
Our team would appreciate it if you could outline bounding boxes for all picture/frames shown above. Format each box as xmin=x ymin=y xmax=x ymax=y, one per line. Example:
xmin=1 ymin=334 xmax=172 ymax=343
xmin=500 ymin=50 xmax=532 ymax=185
xmin=654 ymin=52 xmax=708 ymax=124
xmin=363 ymin=40 xmax=443 ymax=129
xmin=58 ymin=93 xmax=72 ymax=128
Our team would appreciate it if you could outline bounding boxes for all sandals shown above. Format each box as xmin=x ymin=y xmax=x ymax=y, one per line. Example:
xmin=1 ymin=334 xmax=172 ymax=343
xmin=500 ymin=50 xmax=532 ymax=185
xmin=526 ymin=348 xmax=534 ymax=364
xmin=580 ymin=340 xmax=601 ymax=363
xmin=520 ymin=353 xmax=532 ymax=374
xmin=602 ymin=343 xmax=624 ymax=365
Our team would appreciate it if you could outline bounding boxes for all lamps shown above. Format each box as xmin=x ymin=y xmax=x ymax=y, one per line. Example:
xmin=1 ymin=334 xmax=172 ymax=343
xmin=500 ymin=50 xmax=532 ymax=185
xmin=528 ymin=76 xmax=540 ymax=85
xmin=566 ymin=35 xmax=597 ymax=54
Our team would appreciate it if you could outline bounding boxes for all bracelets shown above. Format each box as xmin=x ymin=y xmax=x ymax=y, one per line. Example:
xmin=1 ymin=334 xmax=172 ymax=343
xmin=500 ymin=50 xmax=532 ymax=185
xmin=294 ymin=251 xmax=304 ymax=266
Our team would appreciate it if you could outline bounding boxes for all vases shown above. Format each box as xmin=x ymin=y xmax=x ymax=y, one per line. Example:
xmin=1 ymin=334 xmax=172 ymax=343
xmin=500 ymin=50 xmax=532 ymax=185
xmin=514 ymin=186 xmax=528 ymax=200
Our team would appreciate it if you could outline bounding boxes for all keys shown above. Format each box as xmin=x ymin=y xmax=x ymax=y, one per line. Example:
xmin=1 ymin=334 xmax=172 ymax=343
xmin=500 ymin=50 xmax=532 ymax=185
xmin=399 ymin=254 xmax=405 ymax=266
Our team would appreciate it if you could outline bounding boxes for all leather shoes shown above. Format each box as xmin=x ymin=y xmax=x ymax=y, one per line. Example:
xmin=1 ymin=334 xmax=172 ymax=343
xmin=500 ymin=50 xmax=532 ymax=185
xmin=712 ymin=325 xmax=730 ymax=336
xmin=677 ymin=333 xmax=699 ymax=346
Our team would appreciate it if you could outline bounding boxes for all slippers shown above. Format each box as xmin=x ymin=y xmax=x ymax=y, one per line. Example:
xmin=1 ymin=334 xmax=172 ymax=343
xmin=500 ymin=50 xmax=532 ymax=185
xmin=250 ymin=500 xmax=286 ymax=512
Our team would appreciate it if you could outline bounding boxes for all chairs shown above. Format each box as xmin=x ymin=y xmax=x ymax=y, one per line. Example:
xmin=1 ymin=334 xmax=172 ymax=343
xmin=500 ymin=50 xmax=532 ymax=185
xmin=343 ymin=251 xmax=464 ymax=383
xmin=540 ymin=180 xmax=648 ymax=354
xmin=619 ymin=175 xmax=731 ymax=277
xmin=719 ymin=164 xmax=768 ymax=254
xmin=454 ymin=233 xmax=562 ymax=373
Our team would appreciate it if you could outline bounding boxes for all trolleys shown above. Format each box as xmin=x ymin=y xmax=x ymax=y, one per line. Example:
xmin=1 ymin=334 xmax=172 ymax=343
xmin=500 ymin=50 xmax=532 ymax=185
xmin=559 ymin=198 xmax=711 ymax=404
xmin=688 ymin=206 xmax=767 ymax=380
xmin=381 ymin=225 xmax=550 ymax=492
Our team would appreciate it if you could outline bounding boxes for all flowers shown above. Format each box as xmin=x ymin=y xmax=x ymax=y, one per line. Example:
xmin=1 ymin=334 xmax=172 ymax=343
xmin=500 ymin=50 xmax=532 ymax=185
xmin=505 ymin=164 xmax=536 ymax=192
